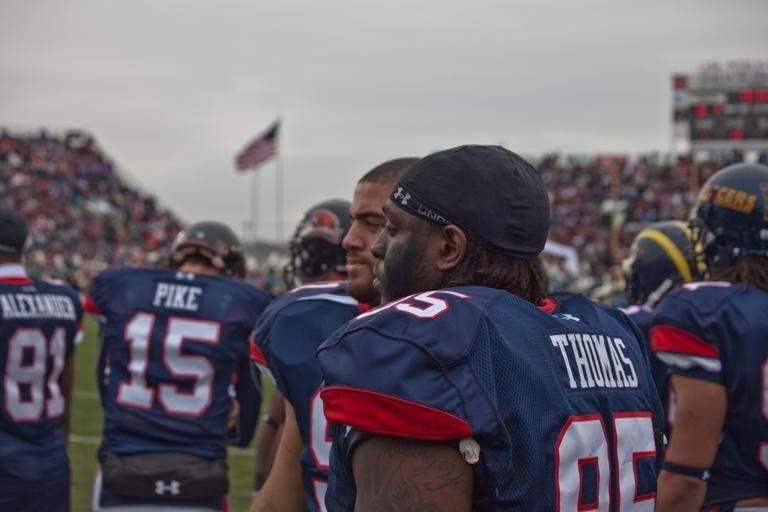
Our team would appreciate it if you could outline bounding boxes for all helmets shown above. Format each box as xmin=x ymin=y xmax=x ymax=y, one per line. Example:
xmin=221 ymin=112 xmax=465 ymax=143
xmin=288 ymin=198 xmax=354 ymax=256
xmin=619 ymin=218 xmax=709 ymax=303
xmin=169 ymin=221 xmax=248 ymax=279
xmin=686 ymin=161 xmax=768 ymax=282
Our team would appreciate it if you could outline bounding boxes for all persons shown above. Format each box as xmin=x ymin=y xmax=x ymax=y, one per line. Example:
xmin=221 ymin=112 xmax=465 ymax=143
xmin=248 ymin=155 xmax=423 ymax=511
xmin=0 ymin=206 xmax=84 ymax=512
xmin=314 ymin=144 xmax=665 ymax=511
xmin=622 ymin=218 xmax=704 ymax=437
xmin=251 ymin=195 xmax=353 ymax=498
xmin=648 ymin=160 xmax=768 ymax=512
xmin=83 ymin=219 xmax=274 ymax=512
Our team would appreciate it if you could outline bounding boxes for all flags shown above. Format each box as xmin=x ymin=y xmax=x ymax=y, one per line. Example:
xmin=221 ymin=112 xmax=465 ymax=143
xmin=235 ymin=124 xmax=277 ymax=172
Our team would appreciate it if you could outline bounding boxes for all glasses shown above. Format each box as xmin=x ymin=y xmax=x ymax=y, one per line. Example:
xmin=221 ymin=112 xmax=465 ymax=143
xmin=296 ymin=241 xmax=349 ymax=277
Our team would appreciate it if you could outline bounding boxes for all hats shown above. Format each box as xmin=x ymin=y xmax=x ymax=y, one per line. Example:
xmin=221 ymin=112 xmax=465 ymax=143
xmin=0 ymin=208 xmax=33 ymax=255
xmin=388 ymin=143 xmax=553 ymax=261
